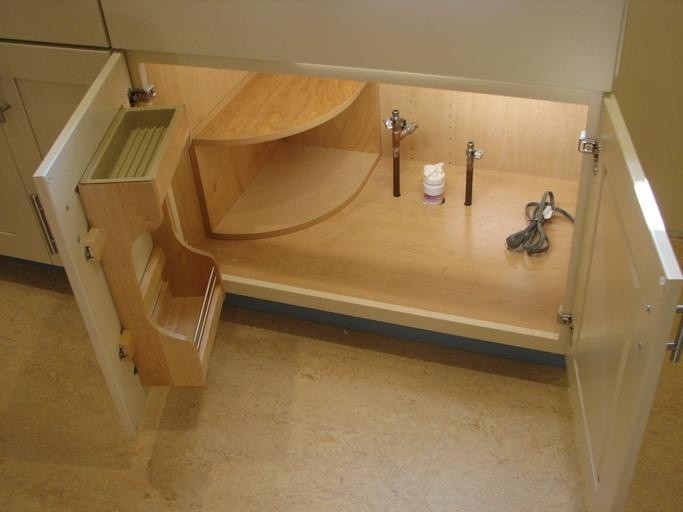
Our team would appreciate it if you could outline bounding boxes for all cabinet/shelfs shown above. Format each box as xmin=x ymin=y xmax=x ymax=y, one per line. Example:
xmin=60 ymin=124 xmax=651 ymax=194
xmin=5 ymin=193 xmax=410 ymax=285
xmin=1 ymin=0 xmax=110 ymax=273
xmin=32 ymin=1 xmax=683 ymax=511
